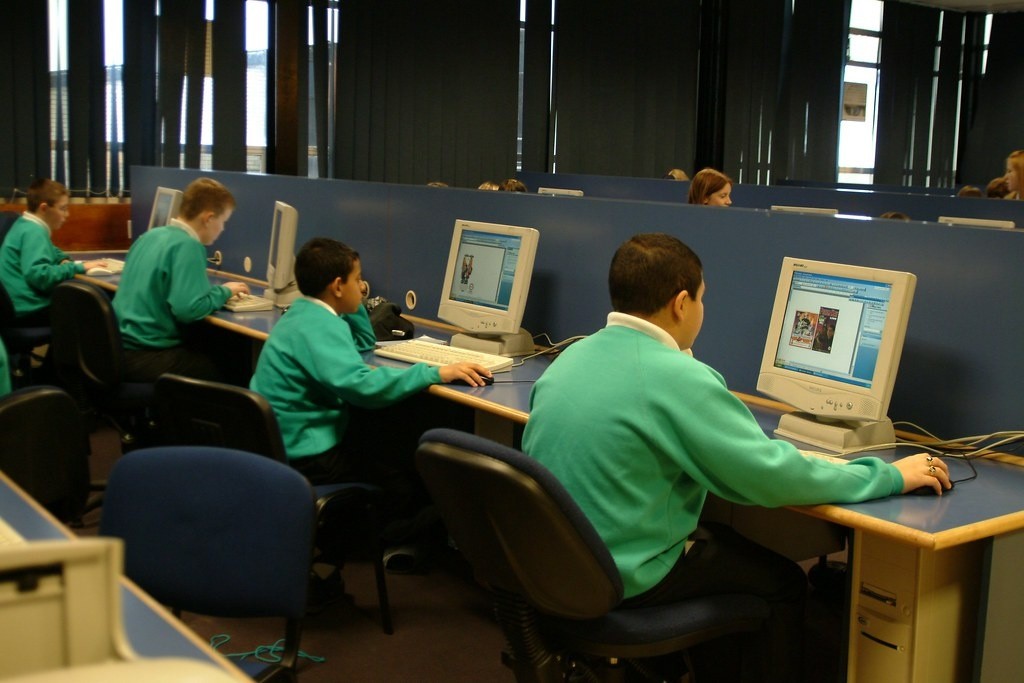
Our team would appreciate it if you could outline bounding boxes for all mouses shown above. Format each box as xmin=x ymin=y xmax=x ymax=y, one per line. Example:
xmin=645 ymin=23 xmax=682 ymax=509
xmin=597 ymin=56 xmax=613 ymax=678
xmin=451 ymin=373 xmax=495 ymax=386
xmin=908 ymin=479 xmax=954 ymax=495
xmin=86 ymin=269 xmax=112 ymax=276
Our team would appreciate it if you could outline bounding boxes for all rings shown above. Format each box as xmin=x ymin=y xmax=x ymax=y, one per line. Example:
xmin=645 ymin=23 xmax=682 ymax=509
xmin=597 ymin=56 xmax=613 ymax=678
xmin=928 ymin=466 xmax=936 ymax=476
xmin=927 ymin=457 xmax=933 ymax=466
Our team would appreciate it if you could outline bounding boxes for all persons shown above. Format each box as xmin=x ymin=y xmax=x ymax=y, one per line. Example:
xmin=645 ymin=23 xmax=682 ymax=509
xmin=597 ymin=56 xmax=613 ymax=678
xmin=668 ymin=169 xmax=688 ymax=179
xmin=958 ymin=151 xmax=1024 ymax=200
xmin=251 ymin=238 xmax=492 ymax=573
xmin=428 ymin=179 xmax=527 ymax=192
xmin=689 ymin=169 xmax=732 ymax=207
xmin=817 ymin=325 xmax=835 ymax=346
xmin=111 ymin=177 xmax=250 ymax=380
xmin=0 ymin=181 xmax=107 ymax=383
xmin=881 ymin=212 xmax=910 ymax=221
xmin=521 ymin=235 xmax=953 ymax=683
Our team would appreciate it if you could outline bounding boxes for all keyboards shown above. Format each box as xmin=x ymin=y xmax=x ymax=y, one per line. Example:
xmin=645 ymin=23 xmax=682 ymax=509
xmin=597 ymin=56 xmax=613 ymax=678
xmin=220 ymin=293 xmax=273 ymax=313
xmin=85 ymin=257 xmax=124 ymax=274
xmin=374 ymin=339 xmax=513 ymax=373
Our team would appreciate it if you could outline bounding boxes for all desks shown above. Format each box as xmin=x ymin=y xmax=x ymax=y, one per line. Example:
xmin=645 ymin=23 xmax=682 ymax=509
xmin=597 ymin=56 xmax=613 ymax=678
xmin=58 ymin=251 xmax=1024 ymax=683
xmin=0 ymin=474 xmax=254 ymax=683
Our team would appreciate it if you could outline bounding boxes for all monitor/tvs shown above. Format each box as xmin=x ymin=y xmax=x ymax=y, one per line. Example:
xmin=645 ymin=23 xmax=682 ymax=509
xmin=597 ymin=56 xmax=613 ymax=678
xmin=263 ymin=201 xmax=304 ymax=307
xmin=437 ymin=218 xmax=540 ymax=356
xmin=147 ymin=186 xmax=184 ymax=232
xmin=756 ymin=256 xmax=917 ymax=453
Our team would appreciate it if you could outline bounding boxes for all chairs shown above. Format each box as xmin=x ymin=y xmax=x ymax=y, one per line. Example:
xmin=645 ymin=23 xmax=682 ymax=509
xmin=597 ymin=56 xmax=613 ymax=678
xmin=0 ymin=282 xmax=56 ymax=389
xmin=108 ymin=443 xmax=318 ymax=681
xmin=416 ymin=429 xmax=772 ymax=681
xmin=161 ymin=370 xmax=392 ymax=639
xmin=49 ymin=281 xmax=156 ymax=456
xmin=0 ymin=386 xmax=108 ymax=528
